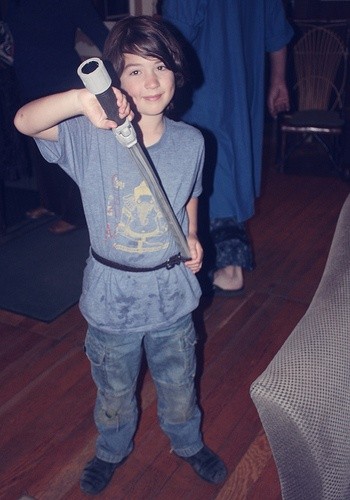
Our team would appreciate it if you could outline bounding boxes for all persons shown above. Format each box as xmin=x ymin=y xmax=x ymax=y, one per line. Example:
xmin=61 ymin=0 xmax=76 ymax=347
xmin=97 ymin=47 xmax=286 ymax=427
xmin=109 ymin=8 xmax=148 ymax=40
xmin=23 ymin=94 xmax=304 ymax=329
xmin=0 ymin=0 xmax=296 ymax=298
xmin=14 ymin=19 xmax=228 ymax=494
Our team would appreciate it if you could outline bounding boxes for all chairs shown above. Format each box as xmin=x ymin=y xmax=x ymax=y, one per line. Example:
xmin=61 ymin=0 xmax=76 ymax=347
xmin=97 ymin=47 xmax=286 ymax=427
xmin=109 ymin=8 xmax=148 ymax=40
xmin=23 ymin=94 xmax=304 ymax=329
xmin=274 ymin=23 xmax=348 ymax=177
xmin=250 ymin=193 xmax=350 ymax=499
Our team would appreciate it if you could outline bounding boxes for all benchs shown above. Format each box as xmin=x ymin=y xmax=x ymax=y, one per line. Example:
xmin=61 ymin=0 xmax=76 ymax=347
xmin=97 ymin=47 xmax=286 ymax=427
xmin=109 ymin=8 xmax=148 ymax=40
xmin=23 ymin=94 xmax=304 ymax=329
xmin=0 ymin=192 xmax=90 ymax=322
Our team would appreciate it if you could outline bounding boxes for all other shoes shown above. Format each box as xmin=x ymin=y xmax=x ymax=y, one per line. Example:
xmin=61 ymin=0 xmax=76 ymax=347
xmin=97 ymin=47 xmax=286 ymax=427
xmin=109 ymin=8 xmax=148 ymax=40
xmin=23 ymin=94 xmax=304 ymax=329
xmin=205 ymin=269 xmax=247 ymax=297
xmin=79 ymin=455 xmax=128 ymax=494
xmin=181 ymin=445 xmax=227 ymax=484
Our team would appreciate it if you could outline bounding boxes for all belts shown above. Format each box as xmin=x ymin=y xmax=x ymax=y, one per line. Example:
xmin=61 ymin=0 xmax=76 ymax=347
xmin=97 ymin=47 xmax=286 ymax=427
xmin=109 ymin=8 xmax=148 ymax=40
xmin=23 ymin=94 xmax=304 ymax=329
xmin=91 ymin=248 xmax=184 ymax=273
